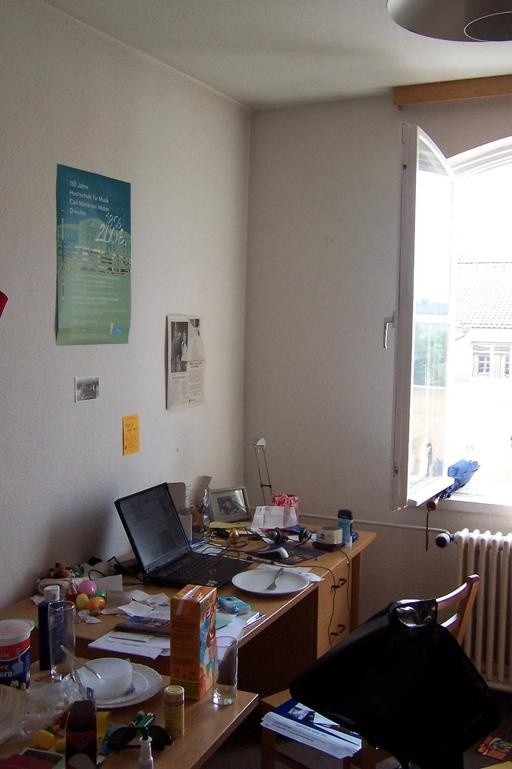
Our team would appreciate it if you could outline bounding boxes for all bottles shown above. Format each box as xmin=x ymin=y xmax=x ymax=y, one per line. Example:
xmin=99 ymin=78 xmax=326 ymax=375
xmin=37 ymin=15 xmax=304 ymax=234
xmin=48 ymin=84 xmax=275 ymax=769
xmin=0 ymin=618 xmax=30 ymax=691
xmin=139 ymin=729 xmax=153 ymax=768
xmin=337 ymin=509 xmax=353 ymax=545
xmin=165 ymin=684 xmax=185 ymax=740
xmin=38 ymin=584 xmax=69 ymax=672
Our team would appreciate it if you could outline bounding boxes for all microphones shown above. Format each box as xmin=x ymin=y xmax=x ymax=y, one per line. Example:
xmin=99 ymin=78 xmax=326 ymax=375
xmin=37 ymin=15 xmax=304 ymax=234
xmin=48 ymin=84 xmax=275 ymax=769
xmin=294 ymin=540 xmax=305 ymax=548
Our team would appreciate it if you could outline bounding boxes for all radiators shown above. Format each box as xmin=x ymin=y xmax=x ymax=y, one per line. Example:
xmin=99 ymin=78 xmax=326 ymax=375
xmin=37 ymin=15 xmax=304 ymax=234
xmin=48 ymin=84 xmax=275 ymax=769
xmin=449 ymin=528 xmax=511 ymax=691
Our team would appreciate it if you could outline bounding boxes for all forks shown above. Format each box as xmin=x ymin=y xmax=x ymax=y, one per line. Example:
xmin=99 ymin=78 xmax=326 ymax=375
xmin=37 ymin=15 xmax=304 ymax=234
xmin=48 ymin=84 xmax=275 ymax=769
xmin=267 ymin=567 xmax=285 ymax=591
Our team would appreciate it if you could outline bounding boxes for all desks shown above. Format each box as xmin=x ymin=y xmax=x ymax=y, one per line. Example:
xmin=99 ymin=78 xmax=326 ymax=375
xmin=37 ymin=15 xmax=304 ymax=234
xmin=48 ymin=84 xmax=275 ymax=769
xmin=0 ymin=516 xmax=377 ymax=687
xmin=4 ymin=655 xmax=258 ymax=768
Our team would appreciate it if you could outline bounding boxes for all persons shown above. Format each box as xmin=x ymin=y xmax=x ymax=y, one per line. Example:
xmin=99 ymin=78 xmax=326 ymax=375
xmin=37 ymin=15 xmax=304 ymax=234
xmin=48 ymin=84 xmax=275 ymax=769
xmin=172 ymin=326 xmax=188 ymax=373
xmin=221 ymin=499 xmax=238 ymax=513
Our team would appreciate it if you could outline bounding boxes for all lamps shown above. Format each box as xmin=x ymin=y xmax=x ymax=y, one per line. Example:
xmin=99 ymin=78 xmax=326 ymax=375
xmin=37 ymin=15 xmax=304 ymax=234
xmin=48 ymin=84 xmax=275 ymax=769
xmin=253 ymin=435 xmax=278 ymax=506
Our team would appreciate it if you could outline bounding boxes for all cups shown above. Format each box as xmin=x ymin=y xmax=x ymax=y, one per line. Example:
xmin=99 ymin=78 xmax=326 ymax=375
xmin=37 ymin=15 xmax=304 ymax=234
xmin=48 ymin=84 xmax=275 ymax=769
xmin=180 ymin=515 xmax=193 ymax=542
xmin=316 ymin=526 xmax=342 ymax=543
xmin=49 ymin=601 xmax=80 ymax=679
xmin=210 ymin=635 xmax=238 ymax=706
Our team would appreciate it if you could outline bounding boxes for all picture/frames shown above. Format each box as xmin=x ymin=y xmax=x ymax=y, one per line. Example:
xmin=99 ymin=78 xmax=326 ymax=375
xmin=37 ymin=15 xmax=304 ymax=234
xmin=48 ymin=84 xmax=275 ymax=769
xmin=208 ymin=487 xmax=251 ymax=522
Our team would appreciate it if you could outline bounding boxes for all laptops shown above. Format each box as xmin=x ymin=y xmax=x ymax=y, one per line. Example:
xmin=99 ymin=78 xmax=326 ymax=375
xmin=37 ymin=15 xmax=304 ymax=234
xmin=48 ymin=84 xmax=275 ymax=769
xmin=114 ymin=482 xmax=252 ymax=591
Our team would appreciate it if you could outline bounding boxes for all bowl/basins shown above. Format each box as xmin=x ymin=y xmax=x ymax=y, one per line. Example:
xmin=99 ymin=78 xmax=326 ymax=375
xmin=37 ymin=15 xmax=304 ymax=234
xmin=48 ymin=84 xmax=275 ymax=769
xmin=77 ymin=656 xmax=135 ymax=700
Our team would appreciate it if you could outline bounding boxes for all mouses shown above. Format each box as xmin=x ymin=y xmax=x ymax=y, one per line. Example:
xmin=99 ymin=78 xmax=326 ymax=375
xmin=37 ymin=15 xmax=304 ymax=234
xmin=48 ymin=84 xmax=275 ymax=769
xmin=257 ymin=546 xmax=289 ymax=558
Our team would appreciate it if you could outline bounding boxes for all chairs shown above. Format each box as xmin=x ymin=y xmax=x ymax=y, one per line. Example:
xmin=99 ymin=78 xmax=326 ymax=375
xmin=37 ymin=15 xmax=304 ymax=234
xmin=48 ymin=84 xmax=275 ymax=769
xmin=260 ymin=573 xmax=482 ymax=768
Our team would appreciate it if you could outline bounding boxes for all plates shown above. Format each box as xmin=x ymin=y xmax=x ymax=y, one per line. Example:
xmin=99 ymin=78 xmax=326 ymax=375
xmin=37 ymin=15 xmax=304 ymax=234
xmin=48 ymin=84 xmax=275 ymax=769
xmin=58 ymin=661 xmax=164 ymax=713
xmin=231 ymin=566 xmax=311 ymax=599
xmin=312 ymin=542 xmax=344 ymax=553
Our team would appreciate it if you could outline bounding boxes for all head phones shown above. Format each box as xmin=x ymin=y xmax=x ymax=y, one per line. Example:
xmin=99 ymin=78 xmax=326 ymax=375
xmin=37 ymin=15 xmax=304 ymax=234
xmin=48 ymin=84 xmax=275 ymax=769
xmin=273 ymin=526 xmax=312 ymax=546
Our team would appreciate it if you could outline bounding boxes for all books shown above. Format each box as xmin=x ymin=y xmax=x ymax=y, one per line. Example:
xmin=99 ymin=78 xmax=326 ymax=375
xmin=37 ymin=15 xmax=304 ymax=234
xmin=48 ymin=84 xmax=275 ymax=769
xmin=115 ymin=614 xmax=170 ymax=639
